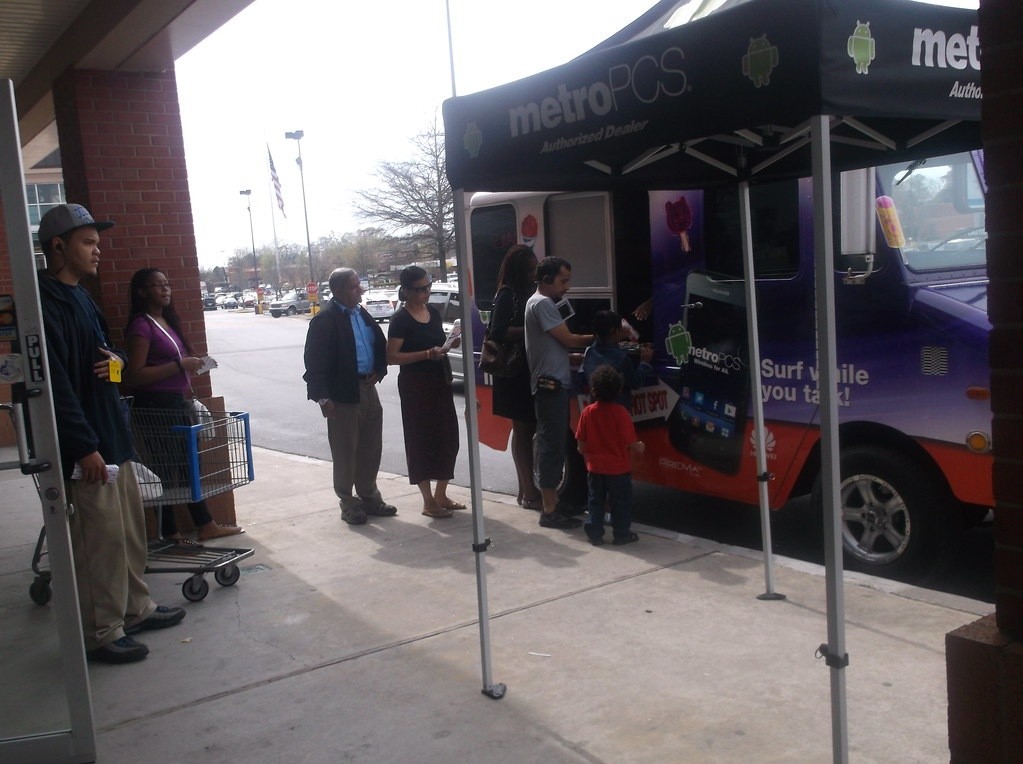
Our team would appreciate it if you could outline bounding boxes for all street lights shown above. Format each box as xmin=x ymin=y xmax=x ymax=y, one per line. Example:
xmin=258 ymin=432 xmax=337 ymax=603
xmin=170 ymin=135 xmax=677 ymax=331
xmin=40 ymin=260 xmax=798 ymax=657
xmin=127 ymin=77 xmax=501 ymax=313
xmin=285 ymin=130 xmax=315 ymax=316
xmin=240 ymin=190 xmax=263 ymax=314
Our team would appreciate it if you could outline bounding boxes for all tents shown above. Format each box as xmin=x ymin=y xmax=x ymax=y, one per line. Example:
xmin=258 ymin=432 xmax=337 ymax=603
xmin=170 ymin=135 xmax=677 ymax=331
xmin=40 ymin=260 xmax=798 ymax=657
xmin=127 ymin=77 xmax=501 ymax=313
xmin=444 ymin=1 xmax=984 ymax=764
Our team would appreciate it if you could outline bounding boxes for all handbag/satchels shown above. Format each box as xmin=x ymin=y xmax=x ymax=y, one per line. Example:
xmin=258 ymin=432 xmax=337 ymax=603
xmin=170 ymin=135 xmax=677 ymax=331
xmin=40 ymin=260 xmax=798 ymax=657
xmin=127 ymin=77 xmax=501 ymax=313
xmin=190 ymin=397 xmax=216 ymax=442
xmin=479 ymin=284 xmax=522 ymax=379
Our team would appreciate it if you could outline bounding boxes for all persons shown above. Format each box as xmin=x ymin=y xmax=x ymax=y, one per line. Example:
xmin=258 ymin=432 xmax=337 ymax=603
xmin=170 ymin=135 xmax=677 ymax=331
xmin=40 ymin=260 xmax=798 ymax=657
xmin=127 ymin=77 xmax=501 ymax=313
xmin=37 ymin=202 xmax=187 ymax=661
xmin=525 ymin=256 xmax=631 ymax=530
xmin=630 ymin=233 xmax=719 ymax=320
xmin=484 ymin=244 xmax=545 ymax=509
xmin=303 ymin=267 xmax=397 ymax=525
xmin=385 ymin=265 xmax=466 ymax=519
xmin=576 ymin=364 xmax=644 ymax=545
xmin=582 ymin=308 xmax=647 ymax=385
xmin=126 ymin=268 xmax=243 ymax=541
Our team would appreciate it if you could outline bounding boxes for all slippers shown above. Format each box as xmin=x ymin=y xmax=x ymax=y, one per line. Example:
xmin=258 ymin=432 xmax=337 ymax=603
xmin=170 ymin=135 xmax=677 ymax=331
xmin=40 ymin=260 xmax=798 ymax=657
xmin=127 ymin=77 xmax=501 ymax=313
xmin=421 ymin=504 xmax=452 ymax=519
xmin=436 ymin=498 xmax=466 ymax=510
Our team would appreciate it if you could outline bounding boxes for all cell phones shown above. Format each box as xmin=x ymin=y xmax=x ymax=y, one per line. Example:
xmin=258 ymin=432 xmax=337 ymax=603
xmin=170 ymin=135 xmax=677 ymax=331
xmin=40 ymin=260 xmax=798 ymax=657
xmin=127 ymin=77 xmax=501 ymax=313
xmin=666 ymin=269 xmax=748 ymax=477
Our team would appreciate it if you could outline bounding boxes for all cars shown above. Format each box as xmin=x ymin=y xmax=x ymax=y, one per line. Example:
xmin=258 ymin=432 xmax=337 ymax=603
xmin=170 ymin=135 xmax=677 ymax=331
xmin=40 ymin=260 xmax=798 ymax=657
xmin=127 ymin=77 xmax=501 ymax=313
xmin=204 ymin=298 xmax=217 ymax=310
xmin=215 ymin=293 xmax=227 ymax=306
xmin=394 ymin=282 xmax=465 ymax=381
xmin=221 ymin=298 xmax=238 ymax=309
xmin=268 ymin=292 xmax=311 ymax=318
xmin=238 ymin=296 xmax=256 ymax=308
xmin=359 ymin=289 xmax=397 ymax=323
xmin=359 ymin=280 xmax=368 ymax=290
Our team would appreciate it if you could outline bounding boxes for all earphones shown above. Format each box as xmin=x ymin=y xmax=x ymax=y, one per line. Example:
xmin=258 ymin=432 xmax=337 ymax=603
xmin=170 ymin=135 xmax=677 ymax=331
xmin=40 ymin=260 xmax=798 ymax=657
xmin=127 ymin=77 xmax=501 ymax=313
xmin=57 ymin=243 xmax=63 ymax=251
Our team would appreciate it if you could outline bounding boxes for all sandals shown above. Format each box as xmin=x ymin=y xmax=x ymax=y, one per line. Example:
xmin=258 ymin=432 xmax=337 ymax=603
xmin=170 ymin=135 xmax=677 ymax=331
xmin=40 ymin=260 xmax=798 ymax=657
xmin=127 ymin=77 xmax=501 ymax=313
xmin=167 ymin=536 xmax=203 ymax=551
xmin=517 ymin=492 xmax=544 ymax=509
xmin=199 ymin=524 xmax=245 ymax=541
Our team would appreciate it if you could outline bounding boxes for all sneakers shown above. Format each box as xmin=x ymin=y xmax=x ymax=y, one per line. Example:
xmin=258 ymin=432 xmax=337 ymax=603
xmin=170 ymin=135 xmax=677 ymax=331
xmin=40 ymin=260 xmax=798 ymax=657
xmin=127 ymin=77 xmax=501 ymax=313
xmin=124 ymin=606 xmax=186 ymax=633
xmin=363 ymin=502 xmax=396 ymax=516
xmin=341 ymin=506 xmax=366 ymax=524
xmin=88 ymin=636 xmax=149 ymax=664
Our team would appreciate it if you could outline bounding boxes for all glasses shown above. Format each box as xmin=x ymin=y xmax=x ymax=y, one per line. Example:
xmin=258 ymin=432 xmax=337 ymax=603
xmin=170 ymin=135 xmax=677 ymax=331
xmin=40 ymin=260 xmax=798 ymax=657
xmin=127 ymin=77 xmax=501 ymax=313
xmin=406 ymin=281 xmax=434 ymax=295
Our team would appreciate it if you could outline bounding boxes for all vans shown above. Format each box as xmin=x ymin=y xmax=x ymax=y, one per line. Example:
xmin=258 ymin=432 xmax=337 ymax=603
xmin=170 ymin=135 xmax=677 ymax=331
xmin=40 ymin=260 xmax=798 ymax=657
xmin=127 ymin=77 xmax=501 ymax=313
xmin=465 ymin=144 xmax=995 ymax=578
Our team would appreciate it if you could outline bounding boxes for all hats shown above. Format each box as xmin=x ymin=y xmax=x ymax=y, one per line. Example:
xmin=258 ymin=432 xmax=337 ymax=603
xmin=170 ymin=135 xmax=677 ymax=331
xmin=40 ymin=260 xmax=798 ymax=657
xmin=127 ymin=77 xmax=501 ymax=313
xmin=38 ymin=203 xmax=116 ymax=246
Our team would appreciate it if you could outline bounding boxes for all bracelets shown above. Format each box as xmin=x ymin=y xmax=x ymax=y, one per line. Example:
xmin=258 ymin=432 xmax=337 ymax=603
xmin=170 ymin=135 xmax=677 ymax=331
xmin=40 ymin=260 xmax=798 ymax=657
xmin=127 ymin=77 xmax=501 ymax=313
xmin=425 ymin=349 xmax=431 ymax=360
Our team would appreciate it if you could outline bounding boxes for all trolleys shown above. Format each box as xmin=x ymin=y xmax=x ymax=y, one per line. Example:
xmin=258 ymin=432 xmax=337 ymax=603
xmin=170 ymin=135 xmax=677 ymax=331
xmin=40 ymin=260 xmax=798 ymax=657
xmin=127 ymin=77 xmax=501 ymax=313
xmin=0 ymin=395 xmax=255 ymax=605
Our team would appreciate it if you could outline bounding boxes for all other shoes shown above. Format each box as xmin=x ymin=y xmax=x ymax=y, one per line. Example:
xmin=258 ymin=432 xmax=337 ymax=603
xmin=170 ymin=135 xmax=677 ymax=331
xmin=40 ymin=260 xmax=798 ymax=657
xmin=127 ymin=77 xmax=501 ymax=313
xmin=539 ymin=507 xmax=583 ymax=529
xmin=613 ymin=534 xmax=639 ymax=545
xmin=587 ymin=536 xmax=605 ymax=546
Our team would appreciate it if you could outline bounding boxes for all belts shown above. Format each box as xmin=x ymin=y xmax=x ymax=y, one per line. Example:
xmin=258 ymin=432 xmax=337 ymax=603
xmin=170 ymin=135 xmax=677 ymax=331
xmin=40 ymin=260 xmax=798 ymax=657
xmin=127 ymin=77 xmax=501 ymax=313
xmin=359 ymin=372 xmax=371 ymax=380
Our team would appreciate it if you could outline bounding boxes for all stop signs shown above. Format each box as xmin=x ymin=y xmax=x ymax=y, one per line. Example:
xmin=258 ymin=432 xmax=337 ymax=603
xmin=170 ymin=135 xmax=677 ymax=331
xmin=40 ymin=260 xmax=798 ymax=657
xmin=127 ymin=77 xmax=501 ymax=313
xmin=306 ymin=283 xmax=318 ymax=294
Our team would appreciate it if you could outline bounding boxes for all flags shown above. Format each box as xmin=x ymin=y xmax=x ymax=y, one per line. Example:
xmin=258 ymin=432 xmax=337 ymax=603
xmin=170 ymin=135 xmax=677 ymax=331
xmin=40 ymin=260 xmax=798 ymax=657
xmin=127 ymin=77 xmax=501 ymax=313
xmin=268 ymin=146 xmax=290 ymax=222
